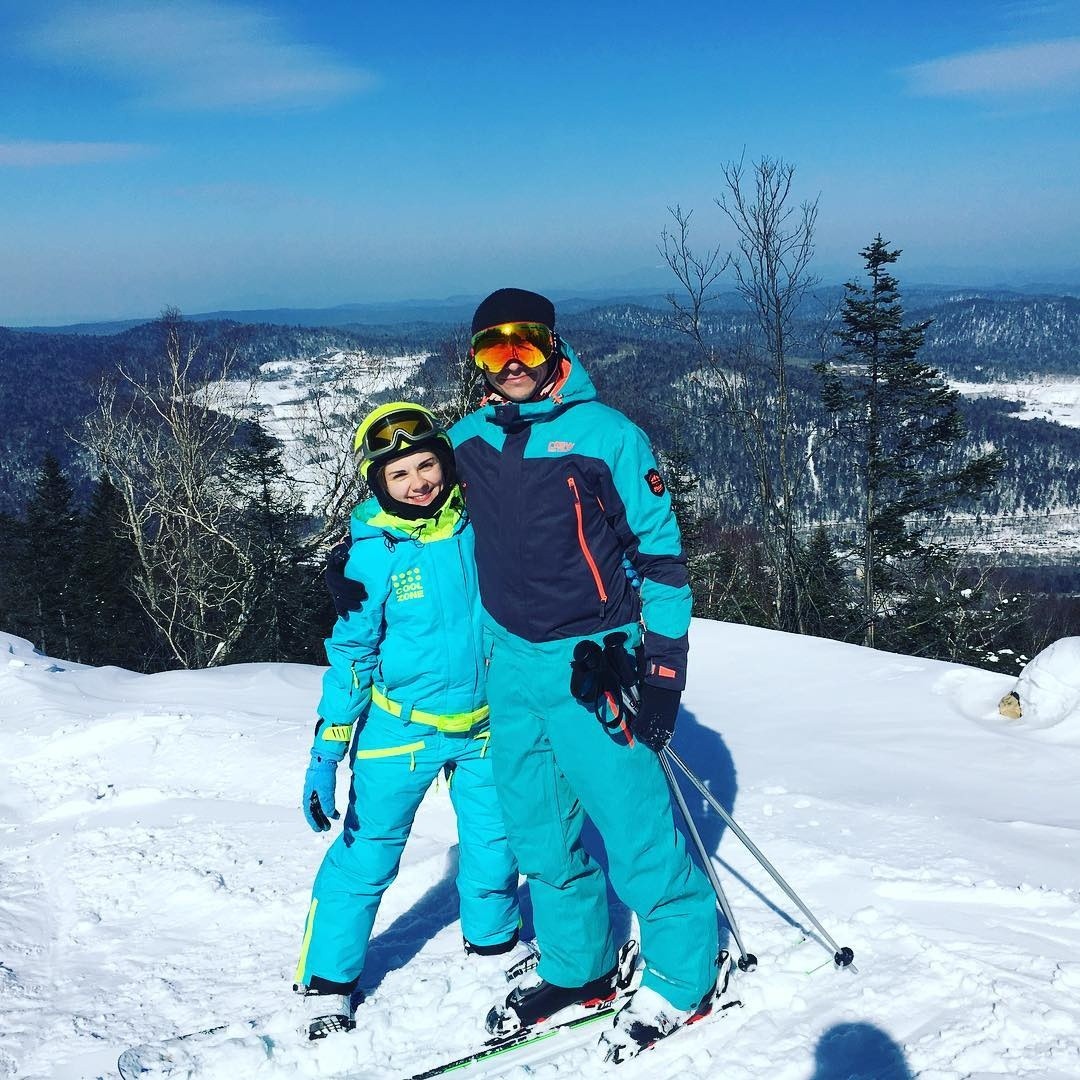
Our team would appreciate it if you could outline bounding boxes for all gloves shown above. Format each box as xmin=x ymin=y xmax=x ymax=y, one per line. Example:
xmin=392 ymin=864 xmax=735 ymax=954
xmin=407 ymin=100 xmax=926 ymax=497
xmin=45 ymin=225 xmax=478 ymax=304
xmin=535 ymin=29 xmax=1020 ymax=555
xmin=327 ymin=541 xmax=368 ymax=616
xmin=634 ymin=683 xmax=680 ymax=753
xmin=620 ymin=554 xmax=640 ymax=588
xmin=303 ymin=758 xmax=340 ymax=832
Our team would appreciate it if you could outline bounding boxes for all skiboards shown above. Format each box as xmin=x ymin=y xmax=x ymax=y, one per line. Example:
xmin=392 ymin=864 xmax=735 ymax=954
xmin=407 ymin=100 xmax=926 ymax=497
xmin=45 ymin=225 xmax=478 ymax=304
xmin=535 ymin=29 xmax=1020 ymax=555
xmin=401 ymin=1008 xmax=617 ymax=1080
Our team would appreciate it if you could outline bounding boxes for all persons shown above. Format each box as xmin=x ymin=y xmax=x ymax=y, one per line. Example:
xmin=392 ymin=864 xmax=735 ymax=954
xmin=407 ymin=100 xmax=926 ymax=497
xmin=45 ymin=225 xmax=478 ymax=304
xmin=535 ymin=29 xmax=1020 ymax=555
xmin=326 ymin=288 xmax=720 ymax=1063
xmin=292 ymin=401 xmax=538 ymax=1040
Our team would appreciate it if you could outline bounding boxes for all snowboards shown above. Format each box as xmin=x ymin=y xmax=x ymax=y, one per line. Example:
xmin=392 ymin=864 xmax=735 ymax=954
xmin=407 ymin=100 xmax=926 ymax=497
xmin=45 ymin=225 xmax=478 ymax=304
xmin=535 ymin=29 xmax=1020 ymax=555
xmin=117 ymin=1005 xmax=310 ymax=1080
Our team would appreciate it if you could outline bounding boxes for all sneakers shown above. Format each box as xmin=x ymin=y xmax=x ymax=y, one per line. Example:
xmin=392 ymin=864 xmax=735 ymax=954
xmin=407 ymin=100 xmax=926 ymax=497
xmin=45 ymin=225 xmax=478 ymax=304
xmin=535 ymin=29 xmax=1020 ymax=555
xmin=614 ymin=980 xmax=717 ymax=1037
xmin=500 ymin=945 xmax=540 ymax=980
xmin=302 ymin=988 xmax=350 ymax=1044
xmin=485 ymin=969 xmax=618 ymax=1037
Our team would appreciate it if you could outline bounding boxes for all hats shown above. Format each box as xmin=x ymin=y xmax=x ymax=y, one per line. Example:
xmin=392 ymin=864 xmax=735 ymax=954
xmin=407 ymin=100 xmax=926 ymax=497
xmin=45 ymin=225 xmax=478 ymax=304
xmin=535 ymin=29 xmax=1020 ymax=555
xmin=471 ymin=289 xmax=555 ymax=333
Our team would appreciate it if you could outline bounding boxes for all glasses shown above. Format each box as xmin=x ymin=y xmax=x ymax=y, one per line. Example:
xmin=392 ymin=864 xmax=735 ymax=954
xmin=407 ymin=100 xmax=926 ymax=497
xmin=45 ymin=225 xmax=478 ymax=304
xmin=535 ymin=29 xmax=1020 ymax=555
xmin=470 ymin=322 xmax=556 ymax=374
xmin=362 ymin=408 xmax=436 ymax=461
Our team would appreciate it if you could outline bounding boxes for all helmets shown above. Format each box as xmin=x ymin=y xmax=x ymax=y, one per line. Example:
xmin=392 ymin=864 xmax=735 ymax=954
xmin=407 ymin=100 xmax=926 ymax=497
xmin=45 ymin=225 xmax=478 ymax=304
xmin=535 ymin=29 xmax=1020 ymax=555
xmin=355 ymin=401 xmax=456 ymax=519
